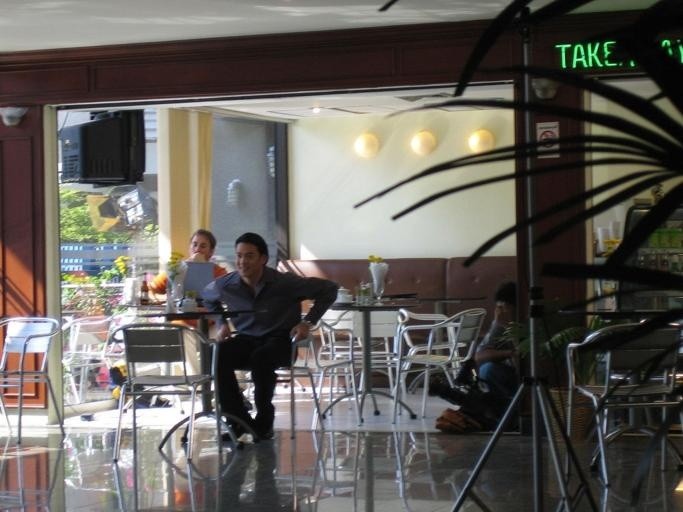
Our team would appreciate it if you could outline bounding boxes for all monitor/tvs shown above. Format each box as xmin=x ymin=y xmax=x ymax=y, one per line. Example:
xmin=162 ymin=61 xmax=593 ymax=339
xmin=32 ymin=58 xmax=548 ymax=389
xmin=59 ymin=109 xmax=146 ymax=188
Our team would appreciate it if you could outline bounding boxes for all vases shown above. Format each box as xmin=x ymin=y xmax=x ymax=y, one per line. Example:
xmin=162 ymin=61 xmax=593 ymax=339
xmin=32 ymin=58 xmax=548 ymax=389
xmin=367 ymin=263 xmax=390 ymax=305
xmin=167 ymin=263 xmax=188 ymax=305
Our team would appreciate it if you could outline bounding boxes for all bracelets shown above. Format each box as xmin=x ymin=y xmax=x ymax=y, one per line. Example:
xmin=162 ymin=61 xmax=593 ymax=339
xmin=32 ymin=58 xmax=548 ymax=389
xmin=302 ymin=319 xmax=314 ymax=330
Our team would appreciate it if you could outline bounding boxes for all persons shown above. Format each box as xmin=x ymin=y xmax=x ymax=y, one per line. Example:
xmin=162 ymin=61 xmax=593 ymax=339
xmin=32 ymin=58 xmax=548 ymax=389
xmin=428 ymin=281 xmax=530 ymax=435
xmin=200 ymin=232 xmax=337 ymax=442
xmin=146 ymin=229 xmax=253 ymax=411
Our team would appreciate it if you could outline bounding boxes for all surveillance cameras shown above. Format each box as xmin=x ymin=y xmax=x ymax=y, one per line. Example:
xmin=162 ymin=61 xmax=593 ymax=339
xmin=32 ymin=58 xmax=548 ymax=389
xmin=1 ymin=108 xmax=30 ymax=126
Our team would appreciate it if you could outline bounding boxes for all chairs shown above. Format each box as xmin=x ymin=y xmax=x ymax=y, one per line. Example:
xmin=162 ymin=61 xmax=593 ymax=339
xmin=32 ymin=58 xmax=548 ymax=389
xmin=563 ymin=322 xmax=683 ymax=512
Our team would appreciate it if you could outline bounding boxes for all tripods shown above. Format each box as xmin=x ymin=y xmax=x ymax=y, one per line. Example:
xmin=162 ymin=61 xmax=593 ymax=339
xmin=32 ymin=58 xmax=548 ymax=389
xmin=448 ymin=6 xmax=598 ymax=512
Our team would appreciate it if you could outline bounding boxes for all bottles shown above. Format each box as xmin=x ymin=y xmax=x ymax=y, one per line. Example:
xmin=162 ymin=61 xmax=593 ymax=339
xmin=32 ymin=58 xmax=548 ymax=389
xmin=173 ymin=271 xmax=184 ymax=309
xmin=141 ymin=272 xmax=149 ymax=305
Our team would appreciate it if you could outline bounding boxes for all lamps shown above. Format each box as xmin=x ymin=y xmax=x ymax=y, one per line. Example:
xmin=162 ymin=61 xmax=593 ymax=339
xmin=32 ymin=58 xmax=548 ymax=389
xmin=354 ymin=129 xmax=495 ymax=158
xmin=0 ymin=107 xmax=29 ymax=127
xmin=531 ymin=78 xmax=557 ymax=98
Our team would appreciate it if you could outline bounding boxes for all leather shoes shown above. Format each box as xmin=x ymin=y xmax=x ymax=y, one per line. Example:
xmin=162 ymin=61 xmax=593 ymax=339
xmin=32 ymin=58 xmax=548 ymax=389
xmin=221 ymin=417 xmax=274 ymax=443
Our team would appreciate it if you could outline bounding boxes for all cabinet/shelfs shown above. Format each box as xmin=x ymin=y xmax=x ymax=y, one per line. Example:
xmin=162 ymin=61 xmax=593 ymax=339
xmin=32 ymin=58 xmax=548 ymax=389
xmin=591 ymin=248 xmax=683 ymax=381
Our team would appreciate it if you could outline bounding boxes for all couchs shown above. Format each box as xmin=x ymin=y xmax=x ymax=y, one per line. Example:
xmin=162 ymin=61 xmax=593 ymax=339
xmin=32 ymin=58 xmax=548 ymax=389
xmin=277 ymin=255 xmax=518 ymax=389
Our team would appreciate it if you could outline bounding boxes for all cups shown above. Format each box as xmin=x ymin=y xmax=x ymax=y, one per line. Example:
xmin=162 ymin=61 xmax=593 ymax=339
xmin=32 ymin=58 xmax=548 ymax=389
xmin=337 ymin=285 xmax=350 ymax=301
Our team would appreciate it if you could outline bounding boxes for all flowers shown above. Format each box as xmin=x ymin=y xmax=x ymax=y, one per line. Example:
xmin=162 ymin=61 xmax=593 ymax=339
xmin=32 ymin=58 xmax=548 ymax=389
xmin=167 ymin=251 xmax=184 ymax=291
xmin=368 ymin=253 xmax=385 ymax=291
